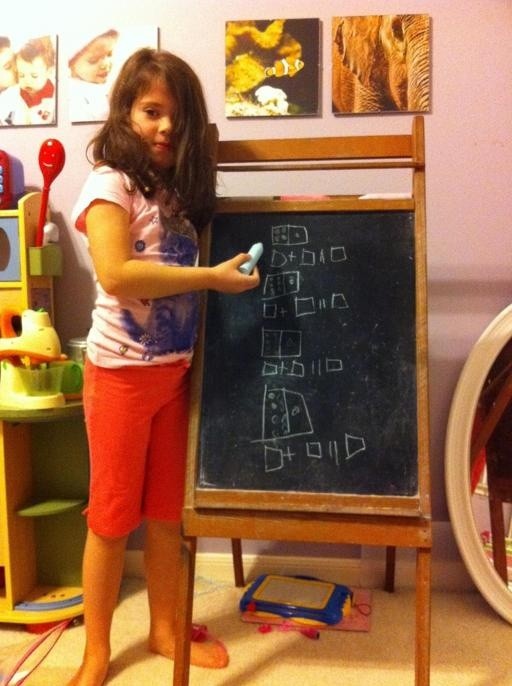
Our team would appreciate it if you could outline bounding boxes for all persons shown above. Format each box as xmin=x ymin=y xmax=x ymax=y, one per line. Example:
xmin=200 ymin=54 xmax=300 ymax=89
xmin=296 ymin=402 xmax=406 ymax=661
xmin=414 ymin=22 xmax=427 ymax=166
xmin=74 ymin=50 xmax=261 ymax=685
xmin=0 ymin=32 xmax=17 ymax=91
xmin=68 ymin=28 xmax=119 ymax=122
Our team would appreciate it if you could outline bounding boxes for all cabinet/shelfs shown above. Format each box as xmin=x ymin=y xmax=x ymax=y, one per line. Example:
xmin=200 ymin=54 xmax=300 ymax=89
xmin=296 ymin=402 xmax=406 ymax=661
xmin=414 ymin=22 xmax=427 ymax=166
xmin=0 ymin=192 xmax=128 ymax=626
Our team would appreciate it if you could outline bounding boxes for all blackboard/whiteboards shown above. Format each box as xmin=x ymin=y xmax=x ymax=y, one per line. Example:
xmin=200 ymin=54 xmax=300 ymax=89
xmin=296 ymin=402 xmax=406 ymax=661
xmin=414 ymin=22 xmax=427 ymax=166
xmin=193 ymin=202 xmax=423 ymax=519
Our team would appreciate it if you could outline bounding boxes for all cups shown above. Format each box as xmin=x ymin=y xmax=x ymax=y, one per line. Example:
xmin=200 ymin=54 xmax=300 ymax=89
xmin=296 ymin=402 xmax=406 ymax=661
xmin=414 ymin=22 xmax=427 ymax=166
xmin=51 ymin=362 xmax=83 ymax=394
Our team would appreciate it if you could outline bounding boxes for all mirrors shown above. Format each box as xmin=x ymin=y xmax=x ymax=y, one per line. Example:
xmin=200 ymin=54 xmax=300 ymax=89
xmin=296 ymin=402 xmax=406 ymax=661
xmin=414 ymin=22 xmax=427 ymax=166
xmin=443 ymin=304 xmax=512 ymax=622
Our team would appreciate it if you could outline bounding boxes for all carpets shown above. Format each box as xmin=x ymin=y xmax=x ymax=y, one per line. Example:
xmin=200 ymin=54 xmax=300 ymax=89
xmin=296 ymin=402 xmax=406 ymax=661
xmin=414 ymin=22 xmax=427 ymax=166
xmin=0 ymin=559 xmax=510 ymax=686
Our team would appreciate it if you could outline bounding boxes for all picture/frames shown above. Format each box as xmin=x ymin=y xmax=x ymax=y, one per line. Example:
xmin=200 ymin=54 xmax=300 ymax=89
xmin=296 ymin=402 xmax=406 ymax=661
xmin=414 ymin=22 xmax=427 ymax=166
xmin=64 ymin=26 xmax=158 ymax=123
xmin=332 ymin=13 xmax=432 ymax=116
xmin=224 ymin=17 xmax=319 ymax=117
